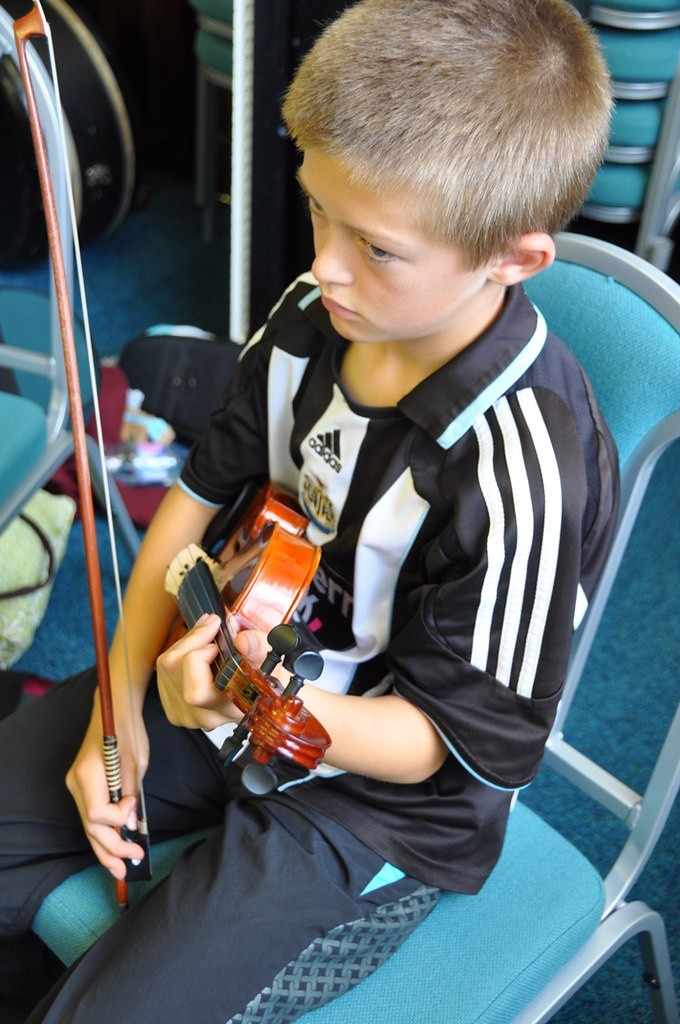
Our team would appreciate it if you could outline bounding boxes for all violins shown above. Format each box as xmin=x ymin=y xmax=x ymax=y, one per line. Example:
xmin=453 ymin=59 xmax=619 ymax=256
xmin=151 ymin=481 xmax=332 ymax=796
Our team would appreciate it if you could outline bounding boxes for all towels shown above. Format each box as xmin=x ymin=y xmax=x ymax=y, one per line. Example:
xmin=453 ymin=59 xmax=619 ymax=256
xmin=0 ymin=487 xmax=79 ymax=672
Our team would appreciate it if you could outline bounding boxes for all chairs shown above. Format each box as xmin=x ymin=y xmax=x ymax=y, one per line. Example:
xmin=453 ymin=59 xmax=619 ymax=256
xmin=0 ymin=0 xmax=680 ymax=1024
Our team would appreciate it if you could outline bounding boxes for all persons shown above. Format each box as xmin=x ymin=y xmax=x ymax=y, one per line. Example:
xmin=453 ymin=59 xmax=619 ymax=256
xmin=1 ymin=1 xmax=622 ymax=1024
